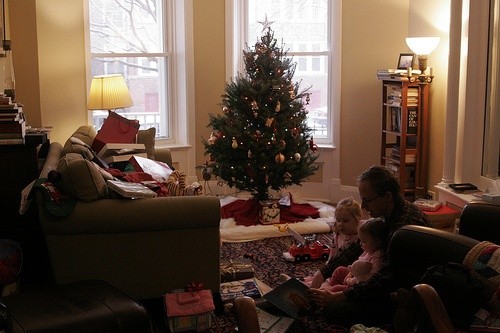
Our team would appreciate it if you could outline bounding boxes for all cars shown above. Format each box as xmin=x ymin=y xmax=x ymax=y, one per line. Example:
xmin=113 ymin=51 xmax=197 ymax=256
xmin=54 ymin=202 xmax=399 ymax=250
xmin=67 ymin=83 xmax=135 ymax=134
xmin=283 ymin=236 xmax=333 ymax=263
xmin=313 ymin=106 xmax=327 ymax=127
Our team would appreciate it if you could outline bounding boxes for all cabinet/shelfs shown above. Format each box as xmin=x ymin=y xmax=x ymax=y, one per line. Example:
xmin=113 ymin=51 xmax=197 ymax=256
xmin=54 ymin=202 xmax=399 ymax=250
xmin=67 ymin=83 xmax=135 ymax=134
xmin=0 ymin=129 xmax=50 ymax=242
xmin=381 ymin=80 xmax=429 ymax=199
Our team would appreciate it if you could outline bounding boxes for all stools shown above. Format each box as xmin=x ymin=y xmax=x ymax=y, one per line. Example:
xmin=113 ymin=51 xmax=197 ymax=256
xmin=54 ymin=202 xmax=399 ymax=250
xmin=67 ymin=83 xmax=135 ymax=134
xmin=0 ymin=278 xmax=147 ymax=333
xmin=421 ymin=204 xmax=460 ymax=228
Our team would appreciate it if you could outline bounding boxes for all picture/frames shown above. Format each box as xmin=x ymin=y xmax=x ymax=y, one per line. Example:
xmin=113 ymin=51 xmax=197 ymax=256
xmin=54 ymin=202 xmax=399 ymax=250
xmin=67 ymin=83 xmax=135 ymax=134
xmin=396 ymin=53 xmax=416 ymax=71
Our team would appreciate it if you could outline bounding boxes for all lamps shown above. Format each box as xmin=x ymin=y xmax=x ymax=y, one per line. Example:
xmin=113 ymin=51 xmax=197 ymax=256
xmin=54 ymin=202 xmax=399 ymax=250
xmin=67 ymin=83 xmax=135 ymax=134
xmin=88 ymin=74 xmax=135 ymax=114
xmin=405 ymin=36 xmax=441 ymax=76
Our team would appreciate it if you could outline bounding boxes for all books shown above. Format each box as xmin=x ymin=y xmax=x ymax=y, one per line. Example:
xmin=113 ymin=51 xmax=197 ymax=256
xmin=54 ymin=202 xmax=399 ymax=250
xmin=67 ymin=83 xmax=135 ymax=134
xmin=413 ymin=198 xmax=443 ymax=212
xmin=263 ymin=277 xmax=319 ymax=322
xmin=0 ymin=103 xmax=25 ymax=144
xmin=221 ymin=281 xmax=263 ymax=302
xmin=386 ymin=88 xmax=419 ymax=189
xmin=234 ymin=307 xmax=280 ymax=333
xmin=107 ymin=180 xmax=157 ymax=198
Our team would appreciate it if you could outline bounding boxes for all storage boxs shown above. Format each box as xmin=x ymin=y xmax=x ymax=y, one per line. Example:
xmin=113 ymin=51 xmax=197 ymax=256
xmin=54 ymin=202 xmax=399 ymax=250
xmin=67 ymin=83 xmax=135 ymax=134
xmin=98 ymin=143 xmax=173 ymax=198
xmin=258 ymin=200 xmax=280 ymax=224
xmin=163 ymin=290 xmax=215 ymax=332
xmin=221 ymin=258 xmax=254 ymax=283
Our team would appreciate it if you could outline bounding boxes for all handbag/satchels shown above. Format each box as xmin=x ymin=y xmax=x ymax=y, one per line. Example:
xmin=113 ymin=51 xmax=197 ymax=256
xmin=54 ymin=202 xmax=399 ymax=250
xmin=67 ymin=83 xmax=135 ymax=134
xmin=91 ymin=106 xmax=140 ymax=154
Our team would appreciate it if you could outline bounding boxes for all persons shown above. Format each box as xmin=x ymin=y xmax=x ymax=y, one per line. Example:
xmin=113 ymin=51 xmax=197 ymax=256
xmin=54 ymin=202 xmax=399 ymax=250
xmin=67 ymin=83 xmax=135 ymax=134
xmin=320 ymin=218 xmax=387 ymax=291
xmin=310 ymin=166 xmax=433 ymax=327
xmin=280 ymin=197 xmax=362 ymax=289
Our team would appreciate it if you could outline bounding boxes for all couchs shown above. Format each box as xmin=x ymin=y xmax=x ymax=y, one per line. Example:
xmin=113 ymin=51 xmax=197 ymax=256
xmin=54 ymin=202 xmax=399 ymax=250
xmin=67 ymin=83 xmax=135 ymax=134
xmin=35 ymin=126 xmax=222 ymax=312
xmin=386 ymin=201 xmax=500 ymax=289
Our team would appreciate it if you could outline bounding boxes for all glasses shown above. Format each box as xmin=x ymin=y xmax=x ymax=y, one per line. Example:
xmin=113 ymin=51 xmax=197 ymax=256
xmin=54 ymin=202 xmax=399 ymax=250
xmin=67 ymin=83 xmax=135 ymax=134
xmin=358 ymin=194 xmax=386 ymax=202
